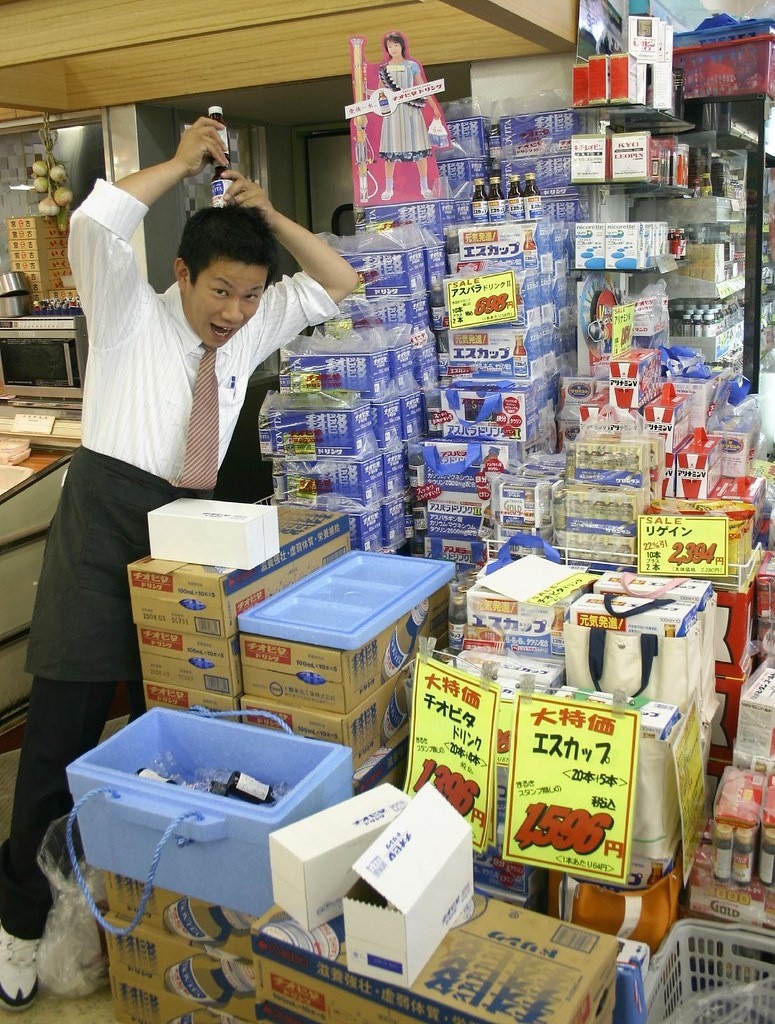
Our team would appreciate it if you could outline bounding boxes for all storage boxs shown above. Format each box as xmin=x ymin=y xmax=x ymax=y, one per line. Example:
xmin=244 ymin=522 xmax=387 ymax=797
xmin=268 ymin=391 xmax=423 ymax=459
xmin=382 ymin=496 xmax=408 ymax=543
xmin=108 ymin=967 xmax=253 ymax=1024
xmin=240 ymin=631 xmax=451 ymax=770
xmin=106 ymin=870 xmax=252 ymax=960
xmin=8 ymin=211 xmax=73 ymax=311
xmin=557 ymin=680 xmax=775 ymax=891
xmin=137 ymin=624 xmax=244 ymax=697
xmin=299 ymin=501 xmax=382 ymax=556
xmin=144 ymin=680 xmax=240 ymax=724
xmin=641 ymin=917 xmax=775 ymax=1024
xmin=382 ymin=449 xmax=408 ymax=500
xmin=275 ymin=16 xmax=775 ymax=401
xmin=416 ymin=401 xmax=775 ymax=678
xmin=475 ymin=824 xmax=542 ymax=898
xmin=285 ymin=461 xmax=384 ymax=503
xmin=250 ymin=894 xmax=618 ymax=1024
xmin=104 ymin=911 xmax=256 ymax=1020
xmin=127 ymin=504 xmax=351 ymax=638
xmin=240 ymin=585 xmax=451 ymax=716
xmin=66 ymin=707 xmax=354 ymax=919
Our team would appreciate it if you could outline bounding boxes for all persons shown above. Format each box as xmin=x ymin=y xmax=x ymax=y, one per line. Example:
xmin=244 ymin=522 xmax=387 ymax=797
xmin=1 ymin=116 xmax=357 ymax=1019
xmin=362 ymin=30 xmax=443 ymax=200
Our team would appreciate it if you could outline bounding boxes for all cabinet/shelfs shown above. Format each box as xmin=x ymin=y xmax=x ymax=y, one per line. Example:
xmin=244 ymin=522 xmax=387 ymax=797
xmin=635 ymin=92 xmax=775 ymax=402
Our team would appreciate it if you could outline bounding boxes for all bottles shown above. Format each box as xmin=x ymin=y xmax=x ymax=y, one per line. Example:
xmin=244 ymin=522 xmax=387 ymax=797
xmin=411 ymin=495 xmax=428 ymax=531
xmin=507 ymin=174 xmax=524 ymax=219
xmin=134 ymin=767 xmax=178 ymax=786
xmin=210 ymin=160 xmax=234 ymax=206
xmin=272 ymin=459 xmax=285 ymax=501
xmin=568 ymin=535 xmax=632 ymax=564
xmin=163 ymin=895 xmax=248 ymax=941
xmin=381 ymin=598 xmax=430 ymax=682
xmin=381 ymin=657 xmax=415 ymax=745
xmin=163 ymin=954 xmax=254 ymax=1005
xmin=445 ymin=230 xmax=460 ymax=275
xmin=258 ymin=406 xmax=271 ymax=458
xmin=554 ymin=485 xmax=569 ymax=532
xmin=448 ymin=569 xmax=480 ymax=655
xmin=569 ymin=494 xmax=634 ymax=521
xmin=207 ymin=106 xmax=230 ymax=162
xmin=513 ymin=335 xmax=528 ymax=377
xmin=404 ymin=498 xmax=415 ymax=538
xmin=487 ymin=177 xmax=505 ymax=223
xmin=723 ymin=252 xmax=745 ymax=280
xmin=551 ymin=607 xmax=565 ymax=654
xmin=430 ymin=283 xmax=445 ymax=330
xmin=477 ymin=500 xmax=493 ymax=541
xmin=427 ymin=396 xmax=443 ymax=437
xmin=408 ymin=446 xmax=427 ymax=487
xmin=165 ymin=1008 xmax=229 ymax=1024
xmin=470 ymin=178 xmax=488 ymax=223
xmin=712 ymin=760 xmax=775 ymax=889
xmin=226 ymin=771 xmax=277 ymax=805
xmin=523 ymin=173 xmax=541 ymax=219
xmin=523 ymin=228 xmax=538 ymax=270
xmin=488 ymin=125 xmax=501 ymax=170
xmin=667 ymin=228 xmax=687 ymax=260
xmin=702 ymin=173 xmax=712 ymax=197
xmin=667 ymin=302 xmax=739 ymax=338
xmin=567 ymin=444 xmax=576 ymax=481
xmin=414 ymin=540 xmax=423 ymax=554
xmin=280 ymin=355 xmax=292 ymax=393
xmin=437 ymin=332 xmax=450 ymax=377
xmin=579 ymin=445 xmax=640 ymax=471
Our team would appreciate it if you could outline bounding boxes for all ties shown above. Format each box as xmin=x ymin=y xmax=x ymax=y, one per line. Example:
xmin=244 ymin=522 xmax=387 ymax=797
xmin=179 ymin=343 xmax=219 ymax=490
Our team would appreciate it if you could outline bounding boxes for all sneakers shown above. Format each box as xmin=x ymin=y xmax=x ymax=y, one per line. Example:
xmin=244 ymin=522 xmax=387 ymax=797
xmin=0 ymin=927 xmax=40 ymax=1012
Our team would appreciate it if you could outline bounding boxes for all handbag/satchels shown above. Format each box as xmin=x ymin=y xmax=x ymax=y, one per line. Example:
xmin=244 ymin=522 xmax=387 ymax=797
xmin=562 ymin=595 xmax=701 ymax=717
xmin=573 ymin=688 xmax=682 ymax=859
xmin=620 ymin=571 xmax=720 ymax=725
xmin=476 ymin=534 xmax=602 ymax=610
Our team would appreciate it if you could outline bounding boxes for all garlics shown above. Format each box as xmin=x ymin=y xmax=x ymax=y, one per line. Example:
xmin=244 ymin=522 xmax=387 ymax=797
xmin=30 ymin=161 xmax=73 ymax=218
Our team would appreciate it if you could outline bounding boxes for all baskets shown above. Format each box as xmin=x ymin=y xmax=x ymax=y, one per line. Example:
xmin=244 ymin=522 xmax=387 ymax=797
xmin=637 ymin=919 xmax=775 ymax=1024
